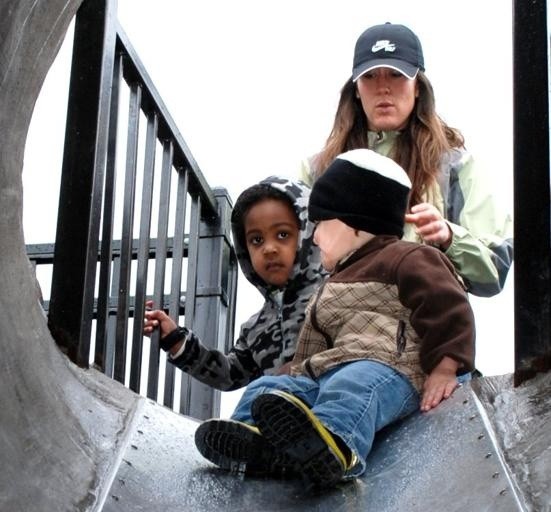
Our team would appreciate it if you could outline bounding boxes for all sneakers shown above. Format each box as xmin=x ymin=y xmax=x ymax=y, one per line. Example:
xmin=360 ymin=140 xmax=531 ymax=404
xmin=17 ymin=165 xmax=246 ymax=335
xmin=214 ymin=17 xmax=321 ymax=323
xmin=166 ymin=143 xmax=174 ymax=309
xmin=194 ymin=418 xmax=299 ymax=479
xmin=250 ymin=388 xmax=348 ymax=492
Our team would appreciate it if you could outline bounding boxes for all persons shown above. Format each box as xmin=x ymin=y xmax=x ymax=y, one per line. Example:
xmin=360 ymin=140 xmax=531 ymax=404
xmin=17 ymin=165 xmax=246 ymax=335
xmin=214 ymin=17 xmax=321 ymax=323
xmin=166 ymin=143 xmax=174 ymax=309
xmin=192 ymin=143 xmax=483 ymax=497
xmin=283 ymin=21 xmax=514 ymax=299
xmin=140 ymin=170 xmax=332 ymax=394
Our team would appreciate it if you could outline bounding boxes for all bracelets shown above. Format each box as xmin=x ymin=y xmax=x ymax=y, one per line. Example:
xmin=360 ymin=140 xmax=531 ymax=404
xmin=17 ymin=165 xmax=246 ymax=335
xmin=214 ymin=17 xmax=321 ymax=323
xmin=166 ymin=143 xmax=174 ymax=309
xmin=159 ymin=327 xmax=187 ymax=350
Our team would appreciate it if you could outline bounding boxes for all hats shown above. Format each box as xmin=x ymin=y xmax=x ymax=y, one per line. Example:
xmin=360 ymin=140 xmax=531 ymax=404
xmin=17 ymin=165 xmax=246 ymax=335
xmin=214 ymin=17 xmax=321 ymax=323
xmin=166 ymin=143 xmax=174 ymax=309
xmin=307 ymin=147 xmax=413 ymax=241
xmin=349 ymin=19 xmax=425 ymax=82
